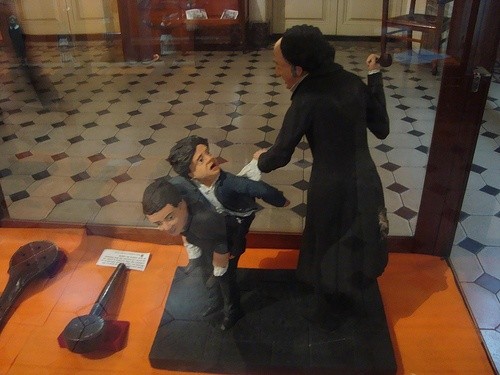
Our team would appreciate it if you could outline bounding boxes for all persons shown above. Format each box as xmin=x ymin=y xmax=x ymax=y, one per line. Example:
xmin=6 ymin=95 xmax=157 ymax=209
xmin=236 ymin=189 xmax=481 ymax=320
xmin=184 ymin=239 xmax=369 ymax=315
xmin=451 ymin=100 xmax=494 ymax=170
xmin=236 ymin=25 xmax=390 ymax=331
xmin=143 ymin=178 xmax=246 ymax=331
xmin=165 ymin=135 xmax=290 ymax=288
xmin=7 ymin=16 xmax=27 ymax=62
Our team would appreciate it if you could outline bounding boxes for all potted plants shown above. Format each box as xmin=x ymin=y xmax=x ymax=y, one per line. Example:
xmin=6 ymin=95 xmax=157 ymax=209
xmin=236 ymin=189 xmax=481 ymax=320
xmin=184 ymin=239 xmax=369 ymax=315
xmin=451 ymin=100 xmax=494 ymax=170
xmin=148 ymin=22 xmax=178 ymax=55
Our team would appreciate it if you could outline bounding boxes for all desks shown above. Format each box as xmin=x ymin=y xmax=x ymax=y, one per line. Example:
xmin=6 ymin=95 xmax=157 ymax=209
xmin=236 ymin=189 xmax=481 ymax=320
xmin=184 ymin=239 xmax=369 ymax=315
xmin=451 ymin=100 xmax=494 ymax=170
xmin=184 ymin=19 xmax=239 ymax=56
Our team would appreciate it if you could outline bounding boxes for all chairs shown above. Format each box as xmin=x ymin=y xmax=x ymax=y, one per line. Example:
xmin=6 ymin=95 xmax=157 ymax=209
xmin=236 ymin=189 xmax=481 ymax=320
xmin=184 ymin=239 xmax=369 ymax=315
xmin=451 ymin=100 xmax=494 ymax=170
xmin=380 ymin=0 xmax=454 ymax=77
